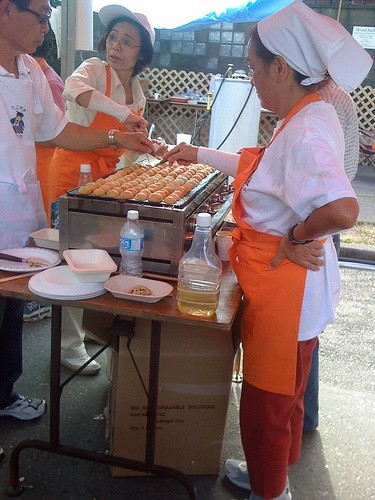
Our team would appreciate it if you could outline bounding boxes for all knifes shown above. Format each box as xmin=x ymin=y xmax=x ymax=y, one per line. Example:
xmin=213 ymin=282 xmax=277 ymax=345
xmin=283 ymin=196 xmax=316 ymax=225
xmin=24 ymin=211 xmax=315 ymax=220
xmin=0 ymin=253 xmax=47 ymax=266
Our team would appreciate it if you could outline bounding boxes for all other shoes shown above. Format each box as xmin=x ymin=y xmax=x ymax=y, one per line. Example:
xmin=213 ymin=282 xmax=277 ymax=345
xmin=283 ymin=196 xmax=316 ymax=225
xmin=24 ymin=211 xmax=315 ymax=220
xmin=245 ymin=476 xmax=292 ymax=500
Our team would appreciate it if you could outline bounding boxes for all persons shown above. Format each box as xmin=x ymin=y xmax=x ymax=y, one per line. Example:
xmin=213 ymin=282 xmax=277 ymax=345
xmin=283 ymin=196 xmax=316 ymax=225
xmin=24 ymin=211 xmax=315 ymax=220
xmin=0 ymin=0 xmax=67 ymax=421
xmin=47 ymin=4 xmax=170 ymax=375
xmin=163 ymin=2 xmax=359 ymax=500
xmin=302 ymin=75 xmax=359 ymax=434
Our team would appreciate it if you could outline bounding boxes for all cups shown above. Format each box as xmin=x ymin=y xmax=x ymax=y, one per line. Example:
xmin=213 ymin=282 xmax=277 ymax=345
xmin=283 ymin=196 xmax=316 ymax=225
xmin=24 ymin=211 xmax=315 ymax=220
xmin=215 ymin=229 xmax=233 ymax=262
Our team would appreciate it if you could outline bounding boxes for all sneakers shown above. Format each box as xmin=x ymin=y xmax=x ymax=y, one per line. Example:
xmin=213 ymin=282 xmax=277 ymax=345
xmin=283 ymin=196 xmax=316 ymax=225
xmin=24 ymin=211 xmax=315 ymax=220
xmin=0 ymin=394 xmax=48 ymax=422
xmin=23 ymin=300 xmax=52 ymax=322
xmin=0 ymin=446 xmax=4 ymax=454
xmin=224 ymin=458 xmax=289 ymax=490
xmin=61 ymin=353 xmax=102 ymax=376
xmin=82 ymin=327 xmax=106 ymax=346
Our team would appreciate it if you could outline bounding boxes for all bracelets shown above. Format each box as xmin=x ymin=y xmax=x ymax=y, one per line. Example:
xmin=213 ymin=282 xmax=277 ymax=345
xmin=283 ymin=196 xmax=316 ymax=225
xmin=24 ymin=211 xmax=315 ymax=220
xmin=288 ymin=223 xmax=315 ymax=246
xmin=107 ymin=129 xmax=120 ymax=146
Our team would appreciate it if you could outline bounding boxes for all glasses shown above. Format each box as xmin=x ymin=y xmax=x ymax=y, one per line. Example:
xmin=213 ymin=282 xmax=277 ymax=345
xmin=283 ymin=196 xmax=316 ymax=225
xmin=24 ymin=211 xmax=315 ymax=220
xmin=23 ymin=6 xmax=52 ymax=25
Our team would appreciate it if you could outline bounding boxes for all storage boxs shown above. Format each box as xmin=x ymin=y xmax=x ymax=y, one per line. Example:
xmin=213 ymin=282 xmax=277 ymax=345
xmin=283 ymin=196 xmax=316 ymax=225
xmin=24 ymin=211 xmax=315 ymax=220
xmin=137 ymin=77 xmax=151 ymax=97
xmin=106 ymin=314 xmax=234 ymax=476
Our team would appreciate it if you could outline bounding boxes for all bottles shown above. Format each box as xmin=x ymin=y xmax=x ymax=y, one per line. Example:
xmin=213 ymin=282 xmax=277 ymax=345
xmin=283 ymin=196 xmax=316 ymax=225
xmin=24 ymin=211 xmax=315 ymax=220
xmin=118 ymin=209 xmax=144 ymax=278
xmin=78 ymin=163 xmax=92 ymax=186
xmin=175 ymin=213 xmax=222 ymax=317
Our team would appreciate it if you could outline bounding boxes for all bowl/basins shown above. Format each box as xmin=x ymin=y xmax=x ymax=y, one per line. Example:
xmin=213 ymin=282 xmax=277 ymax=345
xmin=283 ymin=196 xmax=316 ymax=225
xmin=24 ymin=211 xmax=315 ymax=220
xmin=104 ymin=274 xmax=174 ymax=303
xmin=29 ymin=228 xmax=59 ymax=249
xmin=63 ymin=248 xmax=117 ymax=283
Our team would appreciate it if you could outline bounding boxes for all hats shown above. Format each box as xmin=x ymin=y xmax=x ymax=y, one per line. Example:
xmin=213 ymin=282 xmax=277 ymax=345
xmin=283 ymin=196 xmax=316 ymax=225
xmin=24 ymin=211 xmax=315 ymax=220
xmin=257 ymin=0 xmax=375 ymax=93
xmin=98 ymin=4 xmax=155 ymax=47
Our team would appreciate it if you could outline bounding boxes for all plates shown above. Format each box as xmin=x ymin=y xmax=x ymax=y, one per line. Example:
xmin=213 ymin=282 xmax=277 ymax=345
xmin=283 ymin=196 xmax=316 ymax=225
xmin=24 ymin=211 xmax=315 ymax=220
xmin=27 ymin=264 xmax=110 ymax=300
xmin=170 ymin=97 xmax=191 ymax=102
xmin=0 ymin=249 xmax=62 ymax=271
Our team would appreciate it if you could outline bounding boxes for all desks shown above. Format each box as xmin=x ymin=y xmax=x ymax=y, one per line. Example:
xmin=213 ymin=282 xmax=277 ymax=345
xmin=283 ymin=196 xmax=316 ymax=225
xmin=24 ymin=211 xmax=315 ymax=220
xmin=144 ymin=97 xmax=170 ymax=121
xmin=169 ymin=100 xmax=277 ymax=146
xmin=0 ymin=242 xmax=243 ymax=500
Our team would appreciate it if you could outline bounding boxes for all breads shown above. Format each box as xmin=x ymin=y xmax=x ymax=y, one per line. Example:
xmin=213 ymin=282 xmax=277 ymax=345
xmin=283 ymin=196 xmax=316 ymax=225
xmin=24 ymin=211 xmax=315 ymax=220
xmin=128 ymin=285 xmax=152 ymax=295
xmin=79 ymin=163 xmax=216 ymax=203
